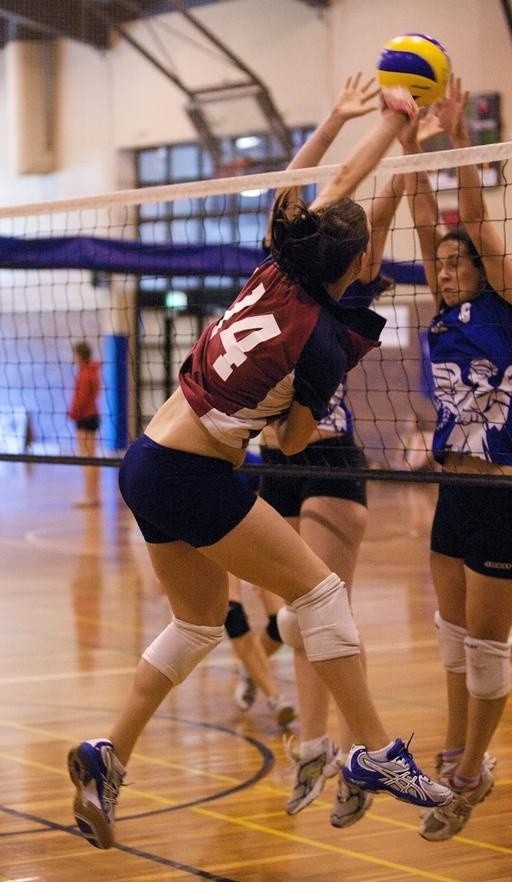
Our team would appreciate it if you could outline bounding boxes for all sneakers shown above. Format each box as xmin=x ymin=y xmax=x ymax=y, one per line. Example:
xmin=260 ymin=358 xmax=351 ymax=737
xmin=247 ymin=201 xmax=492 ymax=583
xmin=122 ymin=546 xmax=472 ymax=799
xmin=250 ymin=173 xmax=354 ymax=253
xmin=68 ymin=737 xmax=129 ymax=849
xmin=233 ymin=661 xmax=298 ymax=727
xmin=285 ymin=737 xmax=497 ymax=842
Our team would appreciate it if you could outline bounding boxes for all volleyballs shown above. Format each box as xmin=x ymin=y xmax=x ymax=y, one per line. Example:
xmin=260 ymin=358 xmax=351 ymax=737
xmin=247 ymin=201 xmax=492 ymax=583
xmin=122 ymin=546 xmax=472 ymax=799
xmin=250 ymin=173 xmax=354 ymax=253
xmin=377 ymin=32 xmax=451 ymax=109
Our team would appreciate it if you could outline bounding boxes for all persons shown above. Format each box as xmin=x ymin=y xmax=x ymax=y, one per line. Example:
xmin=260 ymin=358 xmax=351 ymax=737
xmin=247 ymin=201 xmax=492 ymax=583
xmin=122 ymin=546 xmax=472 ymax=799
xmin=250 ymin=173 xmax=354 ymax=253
xmin=24 ymin=411 xmax=34 ymax=452
